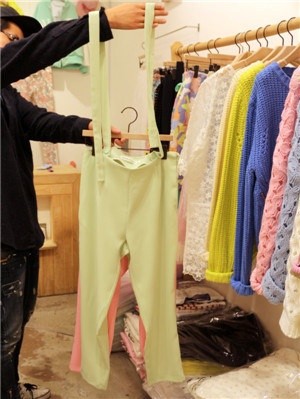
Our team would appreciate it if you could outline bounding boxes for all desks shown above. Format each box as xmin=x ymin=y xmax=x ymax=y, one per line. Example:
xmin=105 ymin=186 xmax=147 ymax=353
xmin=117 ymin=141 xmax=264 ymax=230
xmin=38 ymin=166 xmax=81 ymax=296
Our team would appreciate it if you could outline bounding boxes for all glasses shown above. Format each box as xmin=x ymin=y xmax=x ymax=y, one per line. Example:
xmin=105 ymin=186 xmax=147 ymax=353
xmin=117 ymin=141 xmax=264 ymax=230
xmin=1 ymin=30 xmax=19 ymax=41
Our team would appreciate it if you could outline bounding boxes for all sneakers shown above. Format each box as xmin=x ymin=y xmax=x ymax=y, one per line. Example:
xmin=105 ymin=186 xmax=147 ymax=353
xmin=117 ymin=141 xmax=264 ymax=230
xmin=17 ymin=383 xmax=51 ymax=399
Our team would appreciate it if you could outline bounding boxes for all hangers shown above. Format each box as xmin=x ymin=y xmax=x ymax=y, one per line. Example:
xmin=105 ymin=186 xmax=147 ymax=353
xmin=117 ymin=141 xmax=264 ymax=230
xmin=152 ymin=17 xmax=300 ymax=69
xmin=83 ymin=106 xmax=174 ymax=160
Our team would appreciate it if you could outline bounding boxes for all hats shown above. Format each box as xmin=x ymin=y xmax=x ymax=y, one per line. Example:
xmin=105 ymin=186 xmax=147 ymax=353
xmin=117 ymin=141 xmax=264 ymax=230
xmin=0 ymin=6 xmax=42 ymax=38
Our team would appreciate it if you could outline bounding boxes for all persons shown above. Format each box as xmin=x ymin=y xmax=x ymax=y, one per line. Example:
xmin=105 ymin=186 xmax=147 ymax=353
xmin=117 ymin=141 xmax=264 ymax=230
xmin=0 ymin=3 xmax=168 ymax=399
